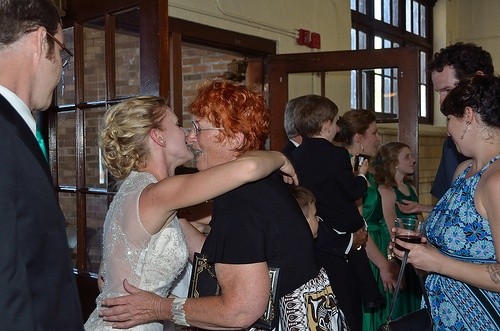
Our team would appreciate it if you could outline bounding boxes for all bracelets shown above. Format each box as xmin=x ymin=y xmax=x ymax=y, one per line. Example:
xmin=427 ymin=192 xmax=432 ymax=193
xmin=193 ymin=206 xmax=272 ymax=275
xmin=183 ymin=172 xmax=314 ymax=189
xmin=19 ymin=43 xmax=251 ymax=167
xmin=171 ymin=297 xmax=190 ymax=327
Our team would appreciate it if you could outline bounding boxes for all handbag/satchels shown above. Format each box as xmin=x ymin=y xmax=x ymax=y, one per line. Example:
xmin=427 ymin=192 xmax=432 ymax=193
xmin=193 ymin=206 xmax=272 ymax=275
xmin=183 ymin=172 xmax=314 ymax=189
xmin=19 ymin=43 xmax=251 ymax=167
xmin=379 ymin=251 xmax=434 ymax=330
xmin=187 ymin=250 xmax=280 ymax=330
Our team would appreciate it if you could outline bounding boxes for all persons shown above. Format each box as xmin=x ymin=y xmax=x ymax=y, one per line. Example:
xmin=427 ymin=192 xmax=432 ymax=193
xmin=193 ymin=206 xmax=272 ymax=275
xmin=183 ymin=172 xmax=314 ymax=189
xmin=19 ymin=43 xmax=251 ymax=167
xmin=372 ymin=142 xmax=427 ymax=320
xmin=389 ymin=74 xmax=500 ymax=331
xmin=289 ymin=95 xmax=387 ymax=310
xmin=282 ymin=99 xmax=363 ymax=331
xmin=292 ymin=186 xmax=318 ymax=239
xmin=99 ymin=77 xmax=351 ymax=330
xmin=429 ymin=43 xmax=494 ymax=199
xmin=83 ymin=94 xmax=298 ymax=331
xmin=334 ymin=109 xmax=404 ymax=331
xmin=0 ymin=0 xmax=86 ymax=331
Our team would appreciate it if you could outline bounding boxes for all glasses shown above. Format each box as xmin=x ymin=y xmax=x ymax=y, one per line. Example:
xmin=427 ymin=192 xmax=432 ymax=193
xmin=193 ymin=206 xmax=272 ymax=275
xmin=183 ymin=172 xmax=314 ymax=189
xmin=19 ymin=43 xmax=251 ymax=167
xmin=24 ymin=27 xmax=73 ymax=67
xmin=190 ymin=122 xmax=225 ymax=136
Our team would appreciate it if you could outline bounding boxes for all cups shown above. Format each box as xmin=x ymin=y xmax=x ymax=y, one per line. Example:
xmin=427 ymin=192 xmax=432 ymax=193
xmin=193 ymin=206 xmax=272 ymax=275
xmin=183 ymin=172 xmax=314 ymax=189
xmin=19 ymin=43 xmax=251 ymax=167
xmin=394 ymin=218 xmax=424 ymax=256
